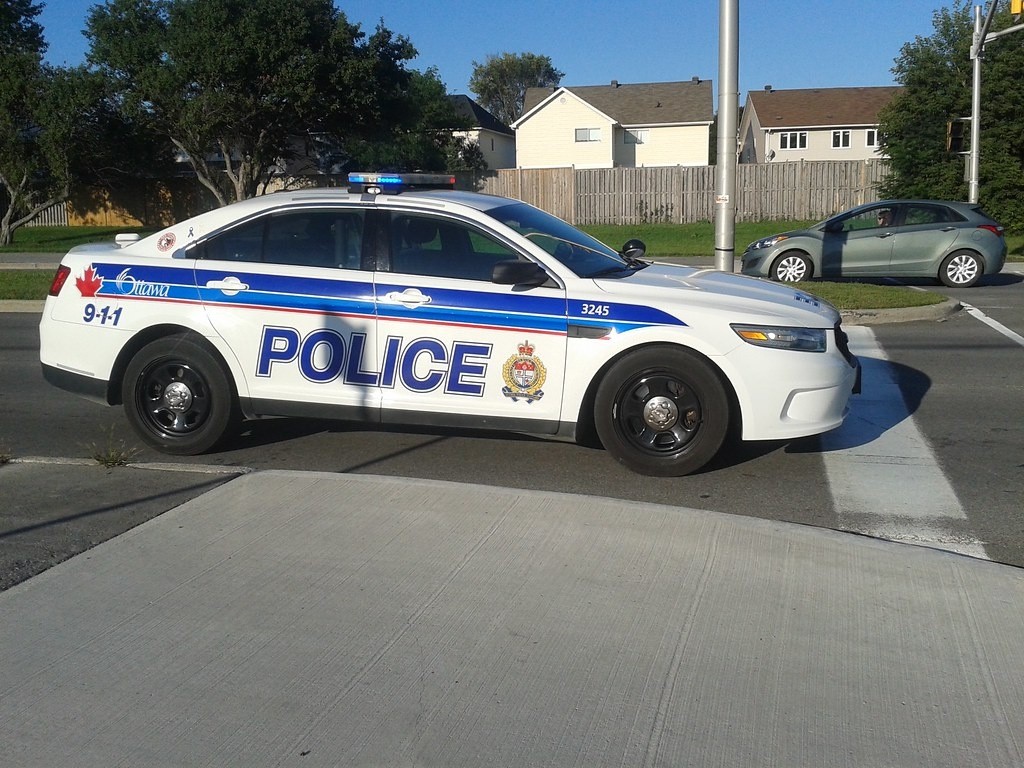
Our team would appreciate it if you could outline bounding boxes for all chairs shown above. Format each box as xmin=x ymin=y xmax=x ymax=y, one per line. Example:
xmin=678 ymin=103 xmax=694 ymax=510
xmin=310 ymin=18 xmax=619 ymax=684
xmin=436 ymin=227 xmax=475 ymax=255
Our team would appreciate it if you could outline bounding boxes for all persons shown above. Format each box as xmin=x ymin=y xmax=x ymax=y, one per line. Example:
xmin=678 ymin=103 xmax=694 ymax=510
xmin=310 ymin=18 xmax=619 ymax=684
xmin=877 ymin=209 xmax=894 ymax=227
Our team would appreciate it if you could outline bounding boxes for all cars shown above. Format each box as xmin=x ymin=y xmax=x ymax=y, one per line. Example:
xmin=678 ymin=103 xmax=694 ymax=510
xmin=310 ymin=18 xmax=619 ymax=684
xmin=35 ymin=168 xmax=861 ymax=480
xmin=745 ymin=196 xmax=1010 ymax=289
